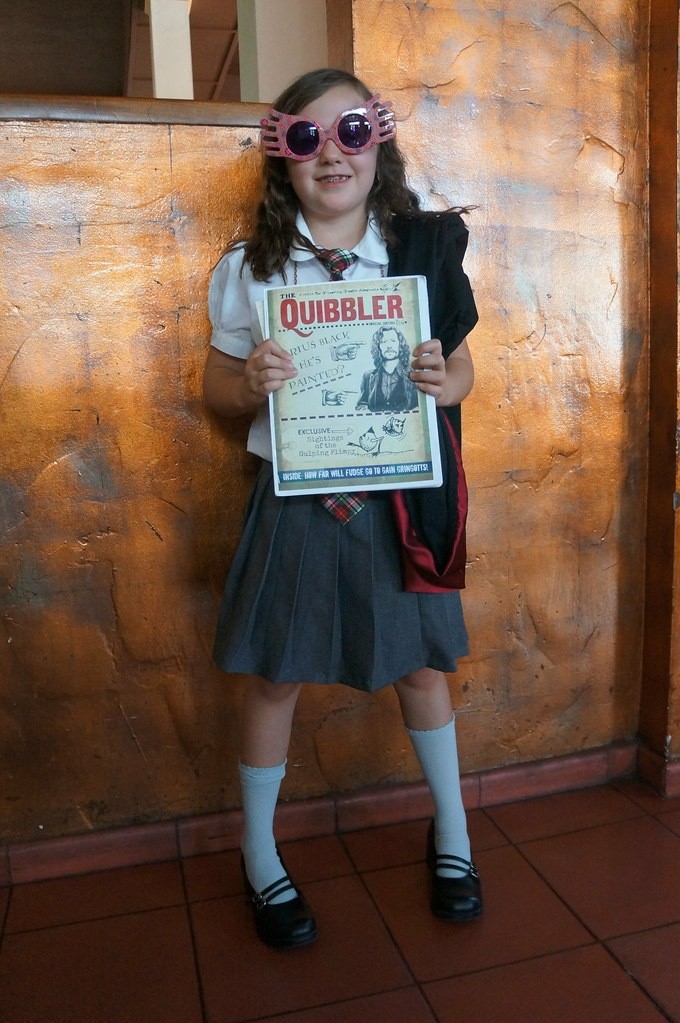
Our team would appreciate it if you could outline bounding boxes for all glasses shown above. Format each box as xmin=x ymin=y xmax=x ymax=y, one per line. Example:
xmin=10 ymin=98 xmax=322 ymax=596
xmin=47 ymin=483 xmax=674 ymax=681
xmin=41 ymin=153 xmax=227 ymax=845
xmin=259 ymin=92 xmax=395 ymax=162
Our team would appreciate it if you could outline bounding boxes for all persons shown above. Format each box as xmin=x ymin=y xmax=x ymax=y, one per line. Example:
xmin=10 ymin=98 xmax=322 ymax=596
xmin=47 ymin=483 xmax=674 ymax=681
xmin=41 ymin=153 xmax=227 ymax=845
xmin=201 ymin=65 xmax=486 ymax=960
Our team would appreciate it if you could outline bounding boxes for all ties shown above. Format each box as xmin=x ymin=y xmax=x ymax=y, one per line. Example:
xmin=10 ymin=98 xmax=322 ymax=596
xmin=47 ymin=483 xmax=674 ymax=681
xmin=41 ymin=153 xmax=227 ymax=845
xmin=293 ymin=231 xmax=370 ymax=525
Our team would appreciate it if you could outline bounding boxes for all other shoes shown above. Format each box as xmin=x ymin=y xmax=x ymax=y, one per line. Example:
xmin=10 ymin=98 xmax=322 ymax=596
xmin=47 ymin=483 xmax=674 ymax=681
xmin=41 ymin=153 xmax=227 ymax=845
xmin=240 ymin=848 xmax=319 ymax=948
xmin=426 ymin=818 xmax=484 ymax=921
